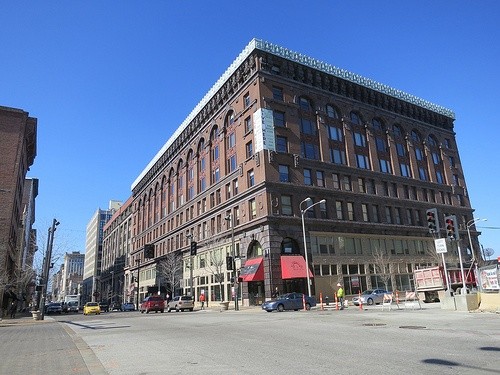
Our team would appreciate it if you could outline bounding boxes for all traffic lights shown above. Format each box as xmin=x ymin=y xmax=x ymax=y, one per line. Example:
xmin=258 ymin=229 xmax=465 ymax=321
xmin=144 ymin=244 xmax=154 ymax=257
xmin=226 ymin=257 xmax=232 ymax=270
xmin=35 ymin=286 xmax=42 ymax=292
xmin=191 ymin=242 xmax=196 ymax=255
xmin=426 ymin=208 xmax=440 ymax=234
xmin=445 ymin=215 xmax=460 ymax=241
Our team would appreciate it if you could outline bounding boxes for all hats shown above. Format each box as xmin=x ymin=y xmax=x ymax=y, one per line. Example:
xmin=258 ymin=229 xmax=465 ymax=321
xmin=337 ymin=283 xmax=341 ymax=286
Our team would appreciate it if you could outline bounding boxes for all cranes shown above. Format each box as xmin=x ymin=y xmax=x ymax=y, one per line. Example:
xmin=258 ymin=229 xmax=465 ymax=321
xmin=63 ymin=295 xmax=79 ymax=313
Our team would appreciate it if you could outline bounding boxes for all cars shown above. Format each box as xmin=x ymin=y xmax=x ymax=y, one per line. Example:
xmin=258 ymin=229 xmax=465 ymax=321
xmin=262 ymin=293 xmax=316 ymax=313
xmin=140 ymin=296 xmax=164 ymax=313
xmin=83 ymin=301 xmax=135 ymax=314
xmin=46 ymin=303 xmax=62 ymax=315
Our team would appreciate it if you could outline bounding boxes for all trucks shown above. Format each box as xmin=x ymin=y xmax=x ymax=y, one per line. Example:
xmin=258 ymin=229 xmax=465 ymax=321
xmin=413 ymin=266 xmax=475 ymax=303
xmin=474 ymin=264 xmax=500 ymax=293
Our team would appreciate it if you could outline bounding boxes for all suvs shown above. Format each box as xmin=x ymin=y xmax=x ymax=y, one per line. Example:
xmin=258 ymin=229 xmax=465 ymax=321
xmin=167 ymin=295 xmax=194 ymax=312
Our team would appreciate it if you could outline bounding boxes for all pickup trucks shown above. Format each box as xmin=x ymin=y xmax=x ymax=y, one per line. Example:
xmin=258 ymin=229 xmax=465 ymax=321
xmin=351 ymin=289 xmax=392 ymax=305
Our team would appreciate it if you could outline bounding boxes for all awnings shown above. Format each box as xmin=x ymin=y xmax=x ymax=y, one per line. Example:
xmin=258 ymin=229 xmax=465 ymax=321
xmin=237 ymin=257 xmax=265 ymax=281
xmin=280 ymin=256 xmax=314 ymax=280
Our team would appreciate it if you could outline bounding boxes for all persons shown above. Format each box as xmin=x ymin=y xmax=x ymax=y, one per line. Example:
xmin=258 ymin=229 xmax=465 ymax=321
xmin=336 ymin=283 xmax=345 ymax=310
xmin=199 ymin=294 xmax=206 ymax=310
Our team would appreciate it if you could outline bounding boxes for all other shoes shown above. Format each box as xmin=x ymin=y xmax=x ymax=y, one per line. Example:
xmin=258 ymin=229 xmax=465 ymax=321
xmin=340 ymin=307 xmax=343 ymax=310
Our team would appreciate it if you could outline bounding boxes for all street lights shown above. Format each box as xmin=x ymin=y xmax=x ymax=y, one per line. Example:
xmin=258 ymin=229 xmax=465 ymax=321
xmin=109 ymin=271 xmax=114 ymax=303
xmin=135 ymin=259 xmax=139 ymax=310
xmin=224 ymin=216 xmax=239 ymax=310
xmin=37 ymin=218 xmax=59 ymax=319
xmin=467 ymin=218 xmax=488 ymax=268
xmin=299 ymin=198 xmax=326 ymax=298
